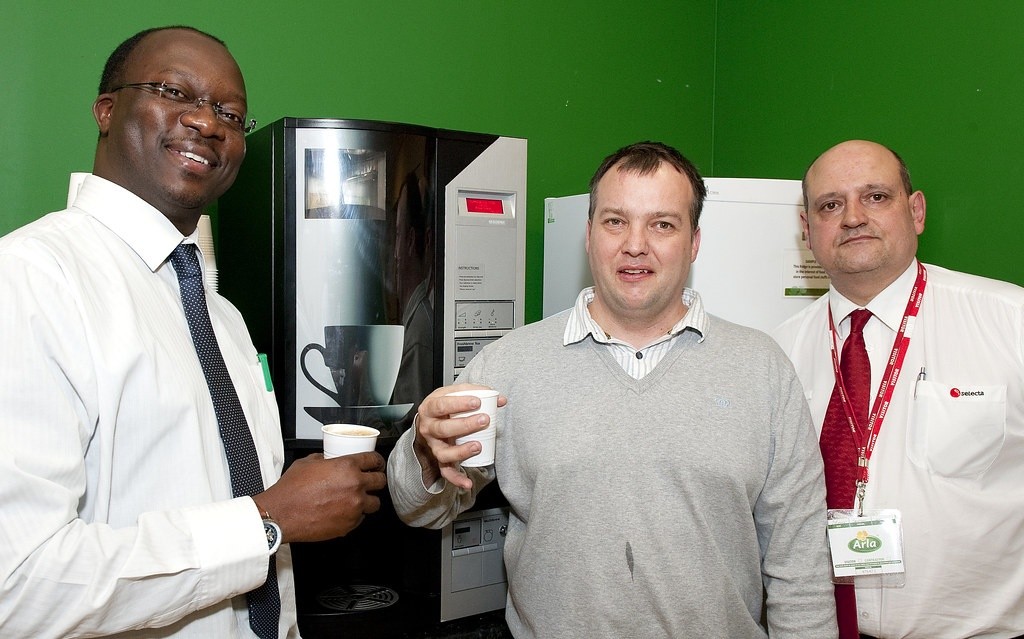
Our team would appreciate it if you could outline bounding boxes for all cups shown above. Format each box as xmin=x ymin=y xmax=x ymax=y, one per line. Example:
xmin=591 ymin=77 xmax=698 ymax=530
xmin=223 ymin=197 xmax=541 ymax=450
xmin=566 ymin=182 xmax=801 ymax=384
xmin=446 ymin=390 xmax=498 ymax=467
xmin=300 ymin=326 xmax=404 ymax=406
xmin=199 ymin=211 xmax=221 ymax=295
xmin=321 ymin=424 xmax=379 ymax=459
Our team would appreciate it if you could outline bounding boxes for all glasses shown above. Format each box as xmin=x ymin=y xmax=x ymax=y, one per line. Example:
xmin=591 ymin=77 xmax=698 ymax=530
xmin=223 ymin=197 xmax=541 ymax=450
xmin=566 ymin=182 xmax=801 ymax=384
xmin=104 ymin=79 xmax=256 ymax=135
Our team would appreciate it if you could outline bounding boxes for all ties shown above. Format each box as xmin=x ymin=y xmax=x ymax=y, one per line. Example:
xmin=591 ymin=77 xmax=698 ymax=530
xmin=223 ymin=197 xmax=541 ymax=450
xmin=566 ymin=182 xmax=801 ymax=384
xmin=168 ymin=243 xmax=281 ymax=639
xmin=820 ymin=310 xmax=869 ymax=639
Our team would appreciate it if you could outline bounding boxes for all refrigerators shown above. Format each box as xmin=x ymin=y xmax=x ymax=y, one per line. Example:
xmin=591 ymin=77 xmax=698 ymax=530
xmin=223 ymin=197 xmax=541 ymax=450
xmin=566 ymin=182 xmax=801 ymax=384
xmin=543 ymin=177 xmax=832 ymax=335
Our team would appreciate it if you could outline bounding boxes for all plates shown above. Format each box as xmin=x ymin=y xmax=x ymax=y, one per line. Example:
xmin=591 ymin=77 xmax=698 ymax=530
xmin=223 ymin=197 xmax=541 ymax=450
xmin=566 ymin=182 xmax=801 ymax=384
xmin=303 ymin=403 xmax=414 ymax=426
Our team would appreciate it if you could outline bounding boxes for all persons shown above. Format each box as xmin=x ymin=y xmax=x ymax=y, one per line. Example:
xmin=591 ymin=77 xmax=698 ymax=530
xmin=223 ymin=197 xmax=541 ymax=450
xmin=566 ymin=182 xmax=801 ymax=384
xmin=768 ymin=138 xmax=1024 ymax=639
xmin=0 ymin=25 xmax=386 ymax=639
xmin=387 ymin=140 xmax=839 ymax=639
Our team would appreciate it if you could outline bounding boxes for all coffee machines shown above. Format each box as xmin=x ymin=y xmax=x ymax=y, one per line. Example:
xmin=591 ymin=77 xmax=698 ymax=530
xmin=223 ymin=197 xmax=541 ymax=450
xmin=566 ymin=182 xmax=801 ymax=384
xmin=219 ymin=116 xmax=527 ymax=639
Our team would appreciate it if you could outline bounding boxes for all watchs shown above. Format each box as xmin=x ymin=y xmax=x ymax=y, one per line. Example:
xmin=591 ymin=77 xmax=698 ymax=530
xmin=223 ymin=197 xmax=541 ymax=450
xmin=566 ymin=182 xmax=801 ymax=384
xmin=255 ymin=502 xmax=282 ymax=561
